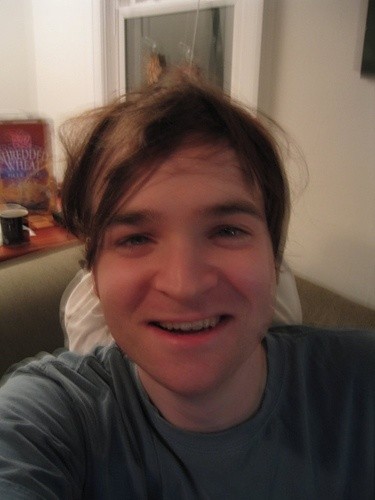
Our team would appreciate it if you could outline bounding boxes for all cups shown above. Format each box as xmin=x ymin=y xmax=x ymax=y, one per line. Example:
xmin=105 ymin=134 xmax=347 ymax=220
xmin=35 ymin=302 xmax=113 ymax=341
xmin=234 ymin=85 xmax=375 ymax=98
xmin=0 ymin=209 xmax=31 ymax=249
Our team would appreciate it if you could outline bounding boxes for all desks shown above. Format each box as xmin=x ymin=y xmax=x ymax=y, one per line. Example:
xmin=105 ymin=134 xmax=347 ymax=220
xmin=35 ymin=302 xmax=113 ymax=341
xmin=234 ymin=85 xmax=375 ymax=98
xmin=0 ymin=212 xmax=79 ymax=264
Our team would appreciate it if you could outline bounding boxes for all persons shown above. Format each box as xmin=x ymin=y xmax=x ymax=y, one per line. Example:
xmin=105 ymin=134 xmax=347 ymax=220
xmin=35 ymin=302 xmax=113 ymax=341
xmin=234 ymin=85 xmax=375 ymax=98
xmin=0 ymin=68 xmax=375 ymax=500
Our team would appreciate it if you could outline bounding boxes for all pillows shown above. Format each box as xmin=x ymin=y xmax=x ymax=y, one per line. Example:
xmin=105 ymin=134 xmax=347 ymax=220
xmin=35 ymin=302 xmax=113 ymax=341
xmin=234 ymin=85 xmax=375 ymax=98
xmin=61 ymin=263 xmax=302 ymax=353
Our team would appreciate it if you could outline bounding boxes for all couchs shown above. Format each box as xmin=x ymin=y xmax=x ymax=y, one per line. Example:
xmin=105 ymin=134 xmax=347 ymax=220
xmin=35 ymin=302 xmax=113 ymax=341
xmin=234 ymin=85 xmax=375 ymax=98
xmin=0 ymin=243 xmax=375 ymax=386
